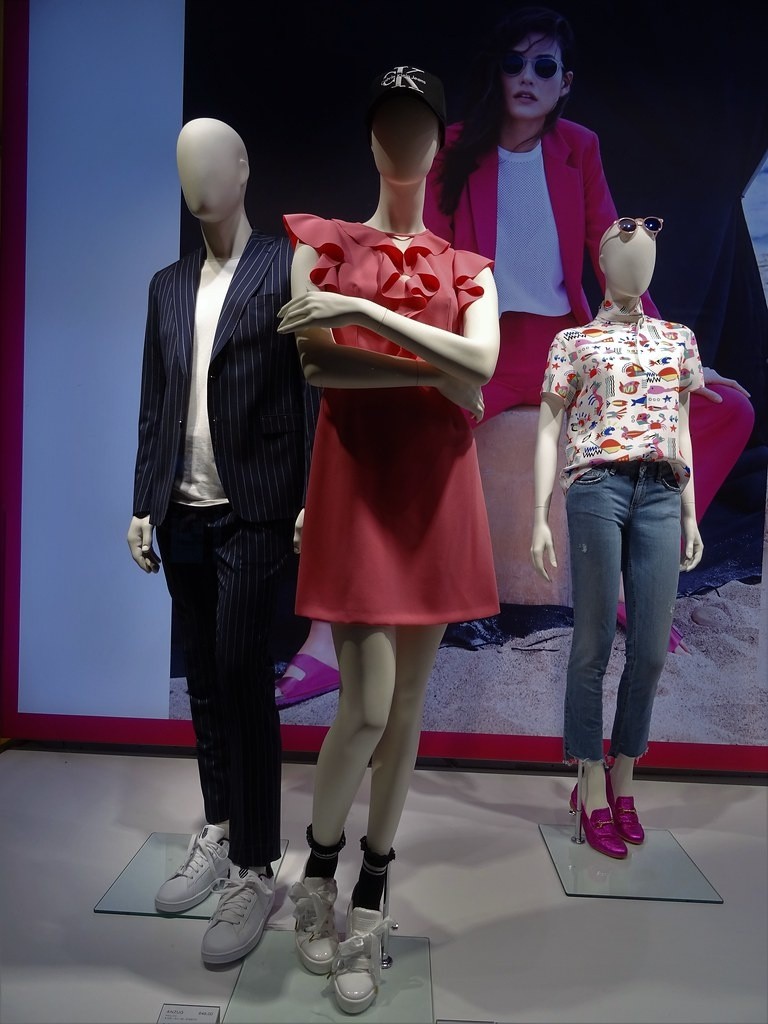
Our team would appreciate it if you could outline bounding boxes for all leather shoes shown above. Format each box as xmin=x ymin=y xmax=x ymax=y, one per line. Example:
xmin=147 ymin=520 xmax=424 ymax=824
xmin=569 ymin=782 xmax=629 ymax=858
xmin=606 ymin=769 xmax=645 ymax=845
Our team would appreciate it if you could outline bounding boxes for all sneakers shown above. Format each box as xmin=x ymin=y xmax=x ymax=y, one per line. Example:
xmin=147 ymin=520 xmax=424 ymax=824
xmin=333 ymin=899 xmax=397 ymax=1014
xmin=287 ymin=857 xmax=340 ymax=975
xmin=154 ymin=824 xmax=229 ymax=912
xmin=200 ymin=865 xmax=276 ymax=964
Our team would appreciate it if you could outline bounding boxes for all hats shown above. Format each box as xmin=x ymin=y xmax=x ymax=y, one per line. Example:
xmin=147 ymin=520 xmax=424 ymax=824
xmin=366 ymin=63 xmax=448 ymax=146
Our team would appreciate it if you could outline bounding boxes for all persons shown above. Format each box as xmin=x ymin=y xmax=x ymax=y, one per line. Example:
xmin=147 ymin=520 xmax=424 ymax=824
xmin=276 ymin=66 xmax=500 ymax=1014
xmin=530 ymin=217 xmax=703 ymax=858
xmin=279 ymin=7 xmax=755 ymax=706
xmin=129 ymin=118 xmax=313 ymax=965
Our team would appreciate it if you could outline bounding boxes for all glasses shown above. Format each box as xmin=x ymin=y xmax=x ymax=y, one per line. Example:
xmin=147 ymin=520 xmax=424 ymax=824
xmin=598 ymin=216 xmax=664 ymax=252
xmin=499 ymin=53 xmax=563 ymax=81
